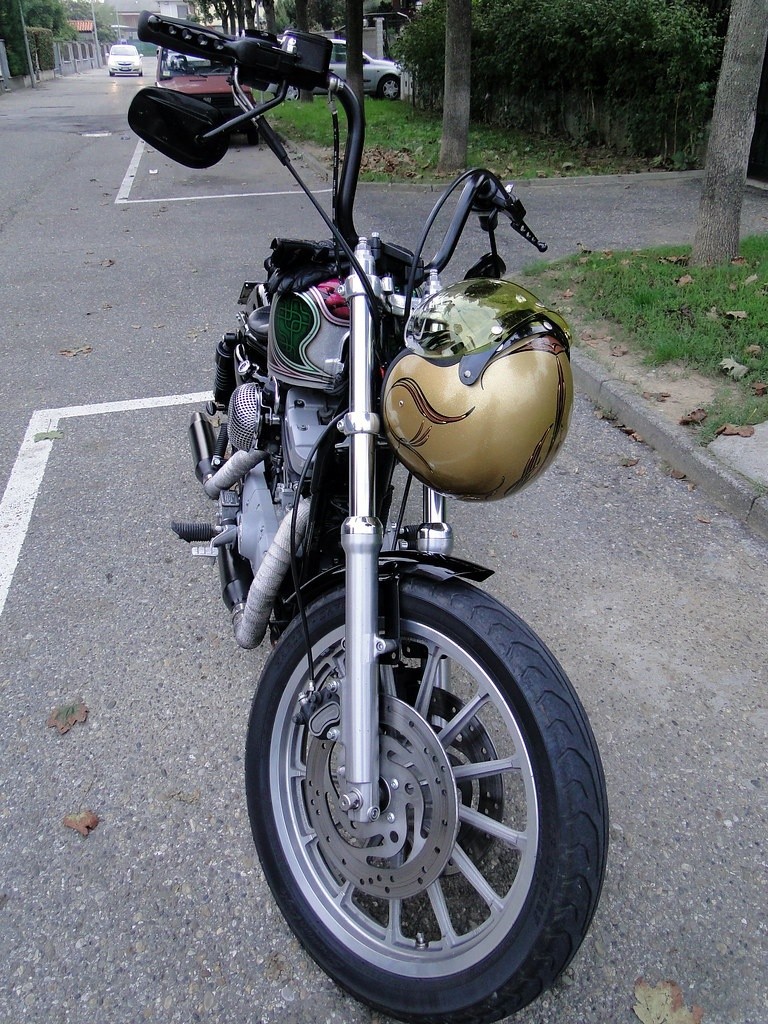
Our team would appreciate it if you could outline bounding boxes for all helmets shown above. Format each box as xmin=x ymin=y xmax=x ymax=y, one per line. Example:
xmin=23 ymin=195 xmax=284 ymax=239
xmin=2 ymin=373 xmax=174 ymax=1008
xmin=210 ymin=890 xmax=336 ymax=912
xmin=380 ymin=276 xmax=575 ymax=504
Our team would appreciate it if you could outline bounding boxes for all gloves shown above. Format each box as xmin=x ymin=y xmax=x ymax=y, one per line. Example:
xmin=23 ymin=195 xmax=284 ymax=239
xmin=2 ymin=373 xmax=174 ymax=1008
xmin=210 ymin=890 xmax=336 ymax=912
xmin=266 ymin=236 xmax=415 ymax=296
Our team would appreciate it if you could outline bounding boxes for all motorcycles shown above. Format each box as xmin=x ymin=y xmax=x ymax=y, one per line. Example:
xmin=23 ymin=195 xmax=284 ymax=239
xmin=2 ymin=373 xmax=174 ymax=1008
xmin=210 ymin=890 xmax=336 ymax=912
xmin=126 ymin=0 xmax=611 ymax=1024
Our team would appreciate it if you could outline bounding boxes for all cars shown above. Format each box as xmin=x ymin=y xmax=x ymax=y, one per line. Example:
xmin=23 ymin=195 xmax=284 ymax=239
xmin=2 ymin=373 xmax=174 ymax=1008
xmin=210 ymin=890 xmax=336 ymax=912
xmin=267 ymin=39 xmax=400 ymax=102
xmin=155 ymin=25 xmax=258 ymax=145
xmin=105 ymin=45 xmax=144 ymax=76
